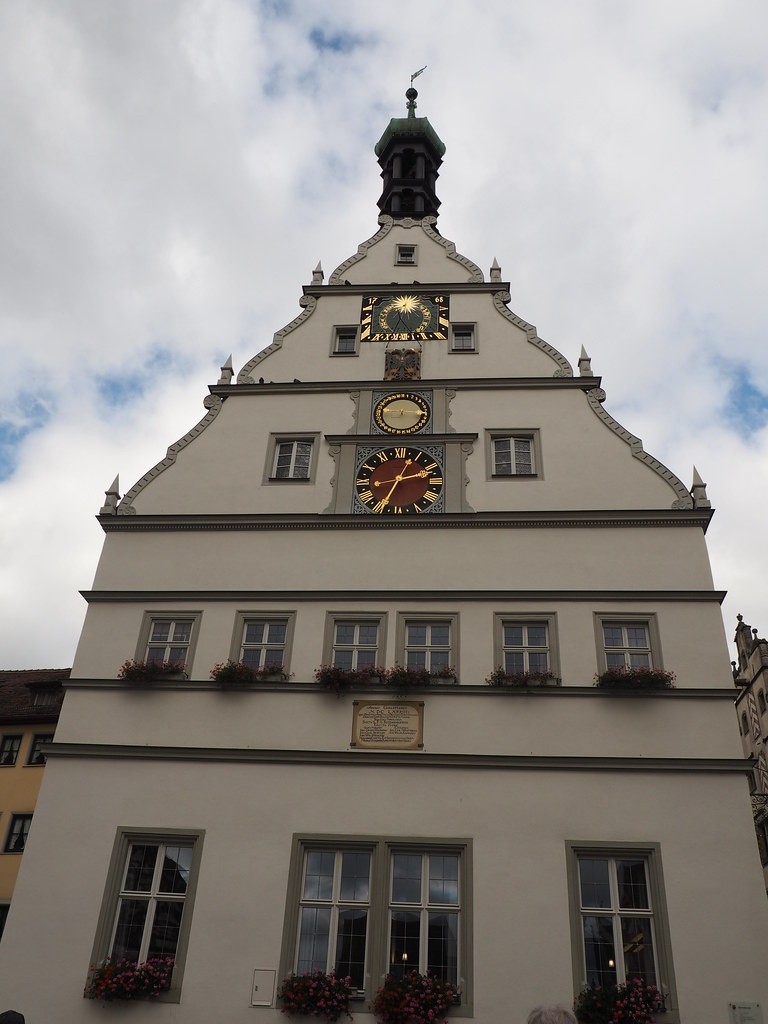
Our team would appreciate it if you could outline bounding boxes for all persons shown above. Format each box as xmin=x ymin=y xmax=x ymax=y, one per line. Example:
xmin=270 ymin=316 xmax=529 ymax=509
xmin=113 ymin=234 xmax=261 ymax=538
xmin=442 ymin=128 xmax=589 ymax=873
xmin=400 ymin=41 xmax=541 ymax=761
xmin=527 ymin=1002 xmax=579 ymax=1024
xmin=0 ymin=1010 xmax=25 ymax=1024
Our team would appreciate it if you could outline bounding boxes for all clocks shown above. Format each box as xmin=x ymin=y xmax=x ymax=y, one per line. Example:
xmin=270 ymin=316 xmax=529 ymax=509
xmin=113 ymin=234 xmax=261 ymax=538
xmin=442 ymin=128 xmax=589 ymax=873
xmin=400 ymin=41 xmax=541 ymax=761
xmin=355 ymin=447 xmax=445 ymax=515
xmin=372 ymin=393 xmax=431 ymax=435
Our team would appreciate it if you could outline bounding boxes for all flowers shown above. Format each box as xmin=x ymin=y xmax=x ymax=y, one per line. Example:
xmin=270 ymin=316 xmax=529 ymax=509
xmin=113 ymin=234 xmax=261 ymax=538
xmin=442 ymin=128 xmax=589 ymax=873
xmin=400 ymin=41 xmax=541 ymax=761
xmin=312 ymin=661 xmax=458 ymax=700
xmin=483 ymin=664 xmax=562 ymax=686
xmin=277 ymin=965 xmax=353 ymax=1024
xmin=83 ymin=956 xmax=175 ymax=1009
xmin=116 ymin=658 xmax=189 ymax=682
xmin=591 ymin=663 xmax=676 ymax=689
xmin=571 ymin=978 xmax=669 ymax=1024
xmin=209 ymin=658 xmax=295 ymax=687
xmin=367 ymin=968 xmax=463 ymax=1024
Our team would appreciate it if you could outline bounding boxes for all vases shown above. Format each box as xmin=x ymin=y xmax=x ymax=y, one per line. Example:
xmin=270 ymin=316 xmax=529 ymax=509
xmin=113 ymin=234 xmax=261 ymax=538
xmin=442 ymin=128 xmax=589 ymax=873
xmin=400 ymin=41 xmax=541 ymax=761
xmin=256 ymin=674 xmax=282 ymax=682
xmin=353 ymin=673 xmax=384 ymax=684
xmin=544 ymin=678 xmax=557 ymax=685
xmin=504 ymin=679 xmax=521 ymax=685
xmin=156 ymin=672 xmax=187 ymax=681
xmin=159 ymin=978 xmax=170 ymax=989
xmin=526 ymin=679 xmax=542 ymax=686
xmin=429 ymin=677 xmax=454 ymax=684
